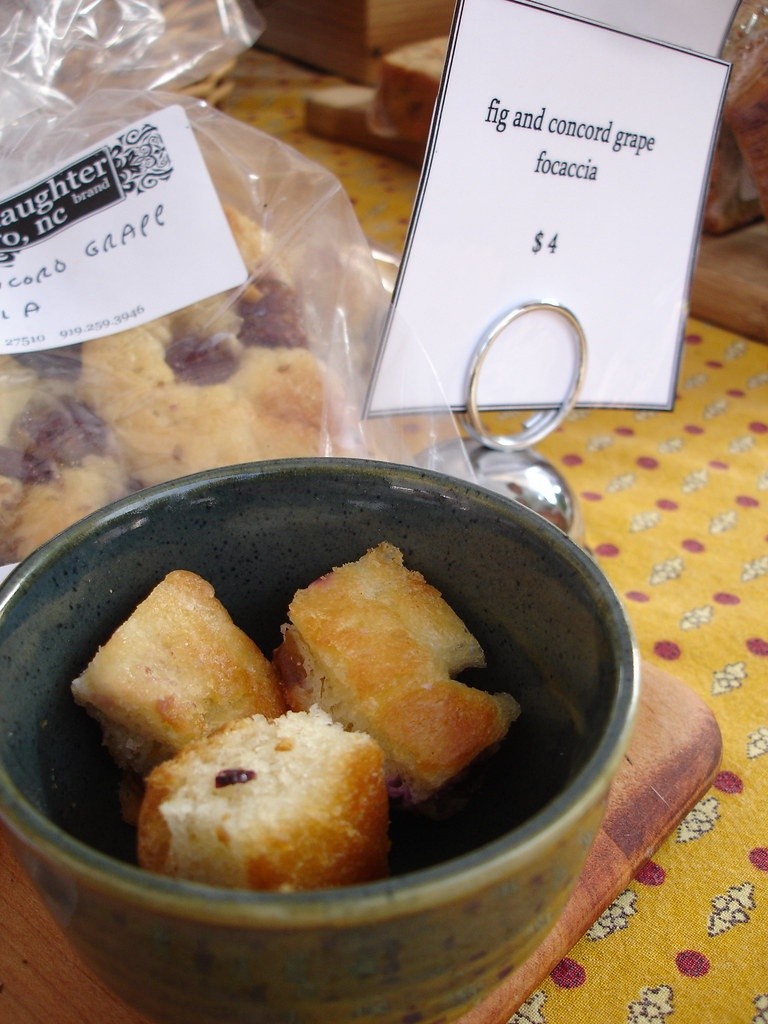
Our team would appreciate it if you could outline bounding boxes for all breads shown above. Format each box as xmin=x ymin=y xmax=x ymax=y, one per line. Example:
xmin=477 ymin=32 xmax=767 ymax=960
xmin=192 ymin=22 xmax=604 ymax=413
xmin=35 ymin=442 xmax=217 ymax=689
xmin=67 ymin=543 xmax=525 ymax=891
xmin=378 ymin=2 xmax=768 ymax=235
xmin=1 ymin=206 xmax=337 ymax=571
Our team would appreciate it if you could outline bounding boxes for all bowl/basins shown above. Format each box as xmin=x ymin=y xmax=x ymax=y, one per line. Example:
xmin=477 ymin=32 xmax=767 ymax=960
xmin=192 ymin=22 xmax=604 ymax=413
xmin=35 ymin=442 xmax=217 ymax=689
xmin=0 ymin=455 xmax=639 ymax=1024
